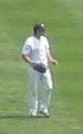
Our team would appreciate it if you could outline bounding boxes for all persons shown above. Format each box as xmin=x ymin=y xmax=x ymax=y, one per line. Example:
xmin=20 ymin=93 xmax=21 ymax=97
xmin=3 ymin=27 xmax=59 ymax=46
xmin=21 ymin=23 xmax=58 ymax=117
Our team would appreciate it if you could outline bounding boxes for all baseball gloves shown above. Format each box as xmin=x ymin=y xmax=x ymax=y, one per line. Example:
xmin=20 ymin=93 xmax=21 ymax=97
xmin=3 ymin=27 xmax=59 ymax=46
xmin=33 ymin=62 xmax=47 ymax=73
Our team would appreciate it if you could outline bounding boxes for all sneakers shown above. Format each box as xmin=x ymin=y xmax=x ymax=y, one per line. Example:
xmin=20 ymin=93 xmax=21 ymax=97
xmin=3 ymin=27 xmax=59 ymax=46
xmin=28 ymin=111 xmax=37 ymax=117
xmin=39 ymin=108 xmax=50 ymax=118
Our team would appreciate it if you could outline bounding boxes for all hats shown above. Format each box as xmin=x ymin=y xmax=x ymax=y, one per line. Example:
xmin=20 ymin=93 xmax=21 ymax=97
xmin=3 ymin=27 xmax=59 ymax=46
xmin=33 ymin=23 xmax=48 ymax=31
